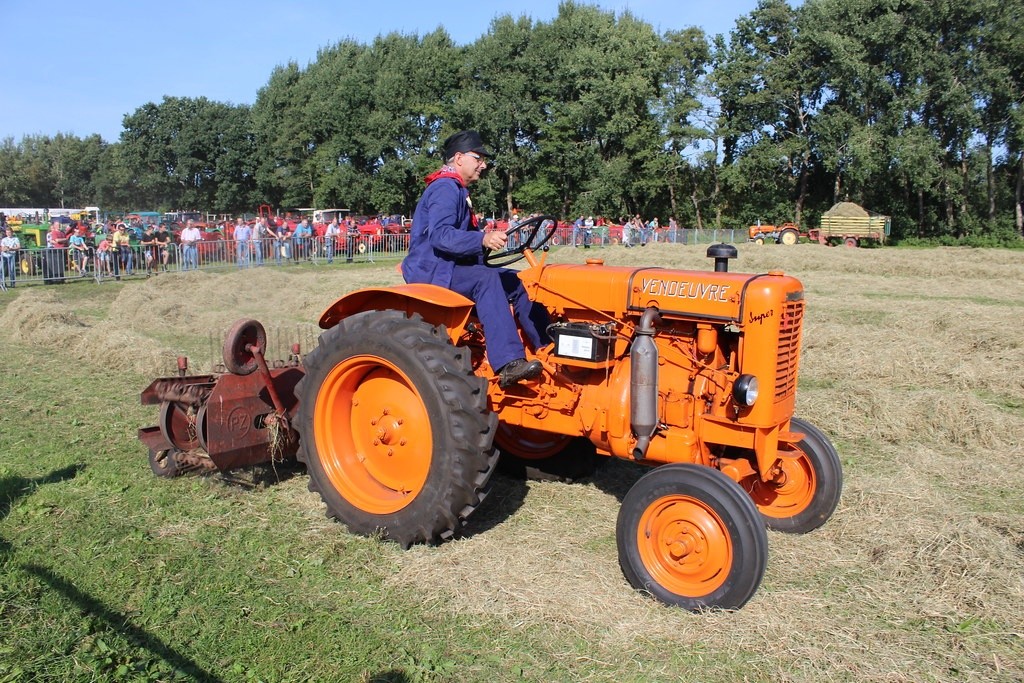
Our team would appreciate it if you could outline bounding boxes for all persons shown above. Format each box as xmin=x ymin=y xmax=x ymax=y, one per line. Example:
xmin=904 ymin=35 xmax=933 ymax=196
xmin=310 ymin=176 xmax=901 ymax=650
xmin=0 ymin=212 xmax=402 ymax=286
xmin=619 ymin=214 xmax=658 ymax=248
xmin=507 ymin=213 xmax=553 ymax=247
xmin=665 ymin=217 xmax=675 ymax=242
xmin=477 ymin=212 xmax=486 ymax=227
xmin=573 ymin=215 xmax=613 ymax=248
xmin=401 ymin=130 xmax=554 ymax=390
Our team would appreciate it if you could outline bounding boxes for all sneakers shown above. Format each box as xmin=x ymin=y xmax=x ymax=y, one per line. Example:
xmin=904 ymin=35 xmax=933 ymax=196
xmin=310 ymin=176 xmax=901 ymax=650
xmin=497 ymin=358 xmax=543 ymax=391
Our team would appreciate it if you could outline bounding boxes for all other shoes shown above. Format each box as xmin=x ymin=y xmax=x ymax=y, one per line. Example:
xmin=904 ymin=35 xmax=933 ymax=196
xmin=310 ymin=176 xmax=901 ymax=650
xmin=79 ymin=269 xmax=86 ymax=276
xmin=162 ymin=264 xmax=168 ymax=271
xmin=146 ymin=274 xmax=151 ymax=279
xmin=126 ymin=271 xmax=135 ymax=275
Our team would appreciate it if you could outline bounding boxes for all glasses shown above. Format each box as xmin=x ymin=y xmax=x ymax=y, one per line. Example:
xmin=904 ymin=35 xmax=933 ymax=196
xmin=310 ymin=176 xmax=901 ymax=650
xmin=448 ymin=153 xmax=487 ymax=163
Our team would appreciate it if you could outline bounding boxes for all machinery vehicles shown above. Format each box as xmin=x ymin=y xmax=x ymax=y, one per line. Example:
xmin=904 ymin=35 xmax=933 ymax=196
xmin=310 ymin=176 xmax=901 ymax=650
xmin=487 ymin=206 xmax=688 ymax=246
xmin=131 ymin=209 xmax=849 ymax=617
xmin=748 ymin=199 xmax=801 ymax=246
xmin=7 ymin=209 xmax=680 ymax=274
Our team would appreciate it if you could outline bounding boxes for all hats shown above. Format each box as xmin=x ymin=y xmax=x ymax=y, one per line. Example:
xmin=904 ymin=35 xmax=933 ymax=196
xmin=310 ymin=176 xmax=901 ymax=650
xmin=444 ymin=130 xmax=493 ymax=160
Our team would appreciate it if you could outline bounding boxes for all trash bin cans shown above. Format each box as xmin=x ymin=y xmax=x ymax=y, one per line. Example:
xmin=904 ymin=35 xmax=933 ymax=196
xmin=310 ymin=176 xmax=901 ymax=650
xmin=41 ymin=248 xmax=65 ymax=284
